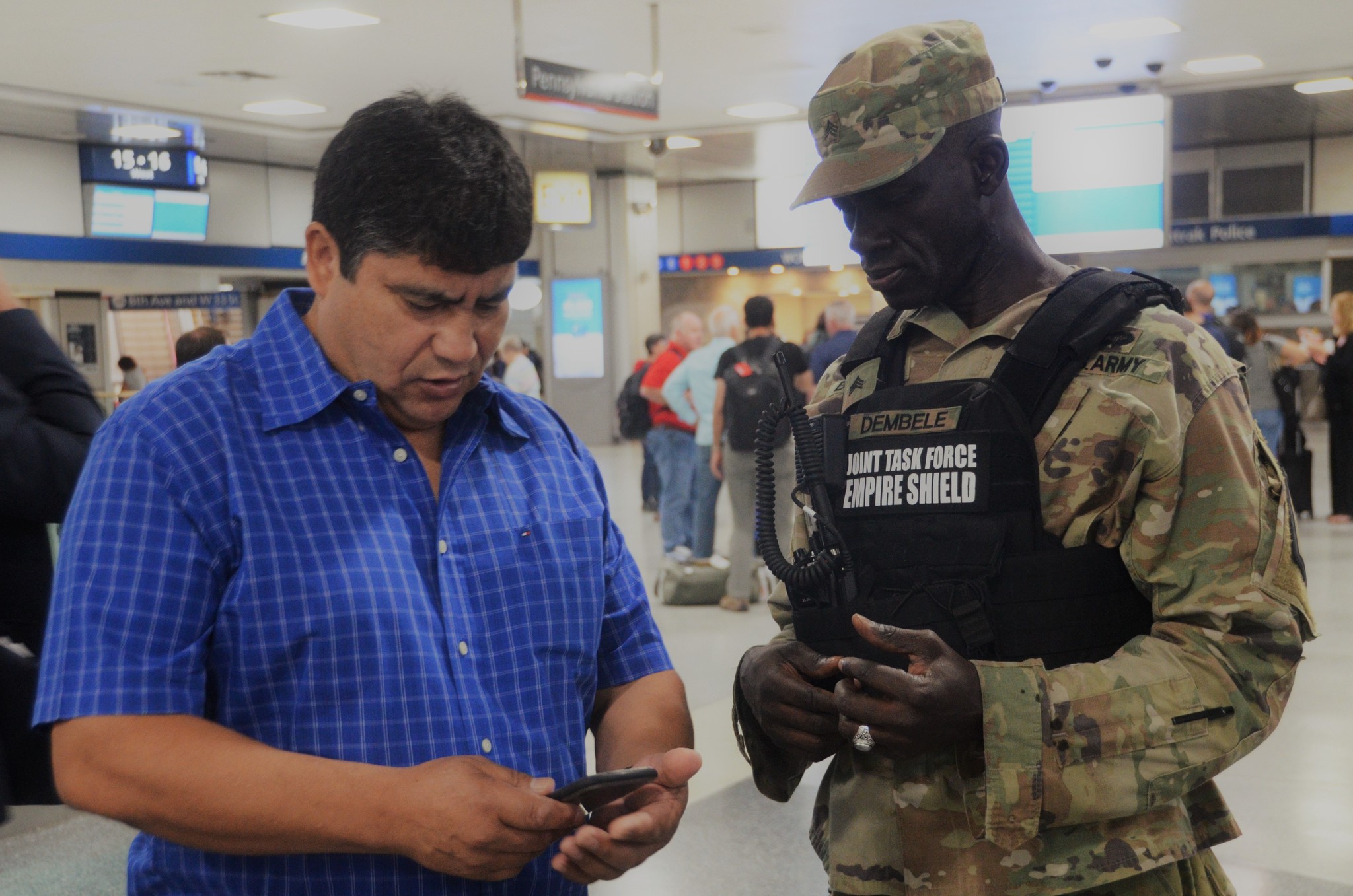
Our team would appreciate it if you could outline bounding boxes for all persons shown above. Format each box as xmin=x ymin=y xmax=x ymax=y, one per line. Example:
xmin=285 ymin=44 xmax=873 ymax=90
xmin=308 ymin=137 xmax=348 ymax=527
xmin=732 ymin=25 xmax=1316 ymax=896
xmin=34 ymin=92 xmax=697 ymax=896
xmin=491 ymin=335 xmax=547 ymax=405
xmin=1171 ymin=277 xmax=1353 ymax=530
xmin=175 ymin=326 xmax=226 ymax=368
xmin=0 ymin=281 xmax=110 ymax=807
xmin=617 ymin=293 xmax=865 ymax=613
xmin=116 ymin=355 xmax=147 ymax=403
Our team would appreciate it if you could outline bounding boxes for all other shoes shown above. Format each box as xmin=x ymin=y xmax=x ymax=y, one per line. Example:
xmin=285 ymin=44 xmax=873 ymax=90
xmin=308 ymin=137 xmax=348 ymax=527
xmin=720 ymin=595 xmax=750 ymax=614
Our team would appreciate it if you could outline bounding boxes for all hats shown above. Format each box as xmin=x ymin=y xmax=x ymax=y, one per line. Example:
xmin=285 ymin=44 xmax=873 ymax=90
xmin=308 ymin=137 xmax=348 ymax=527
xmin=789 ymin=23 xmax=1007 ymax=210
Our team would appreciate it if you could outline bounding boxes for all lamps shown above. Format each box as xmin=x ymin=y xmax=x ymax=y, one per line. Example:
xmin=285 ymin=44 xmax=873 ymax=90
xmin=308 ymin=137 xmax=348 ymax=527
xmin=1294 ymin=76 xmax=1353 ymax=94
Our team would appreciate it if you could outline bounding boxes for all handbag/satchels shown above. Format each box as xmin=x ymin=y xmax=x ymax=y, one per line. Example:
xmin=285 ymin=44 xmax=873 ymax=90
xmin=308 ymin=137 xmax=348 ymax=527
xmin=1272 ymin=366 xmax=1300 ymax=417
xmin=659 ymin=557 xmax=761 ymax=607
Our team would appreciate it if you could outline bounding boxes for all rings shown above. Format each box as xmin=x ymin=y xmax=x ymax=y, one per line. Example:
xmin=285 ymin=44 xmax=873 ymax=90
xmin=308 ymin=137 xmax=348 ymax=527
xmin=853 ymin=725 xmax=876 ymax=753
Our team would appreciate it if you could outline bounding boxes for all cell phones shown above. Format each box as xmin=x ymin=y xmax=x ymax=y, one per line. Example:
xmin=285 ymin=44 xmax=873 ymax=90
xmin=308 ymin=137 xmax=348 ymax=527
xmin=546 ymin=766 xmax=659 ymax=812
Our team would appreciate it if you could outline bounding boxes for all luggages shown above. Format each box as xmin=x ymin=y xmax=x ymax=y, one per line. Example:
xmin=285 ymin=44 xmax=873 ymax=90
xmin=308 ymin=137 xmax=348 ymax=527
xmin=1281 ymin=451 xmax=1314 ymax=519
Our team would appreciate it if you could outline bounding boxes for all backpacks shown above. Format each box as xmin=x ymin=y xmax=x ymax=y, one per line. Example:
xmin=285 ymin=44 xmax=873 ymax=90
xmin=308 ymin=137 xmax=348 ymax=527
xmin=723 ymin=337 xmax=793 ymax=452
xmin=615 ymin=364 xmax=653 ymax=439
